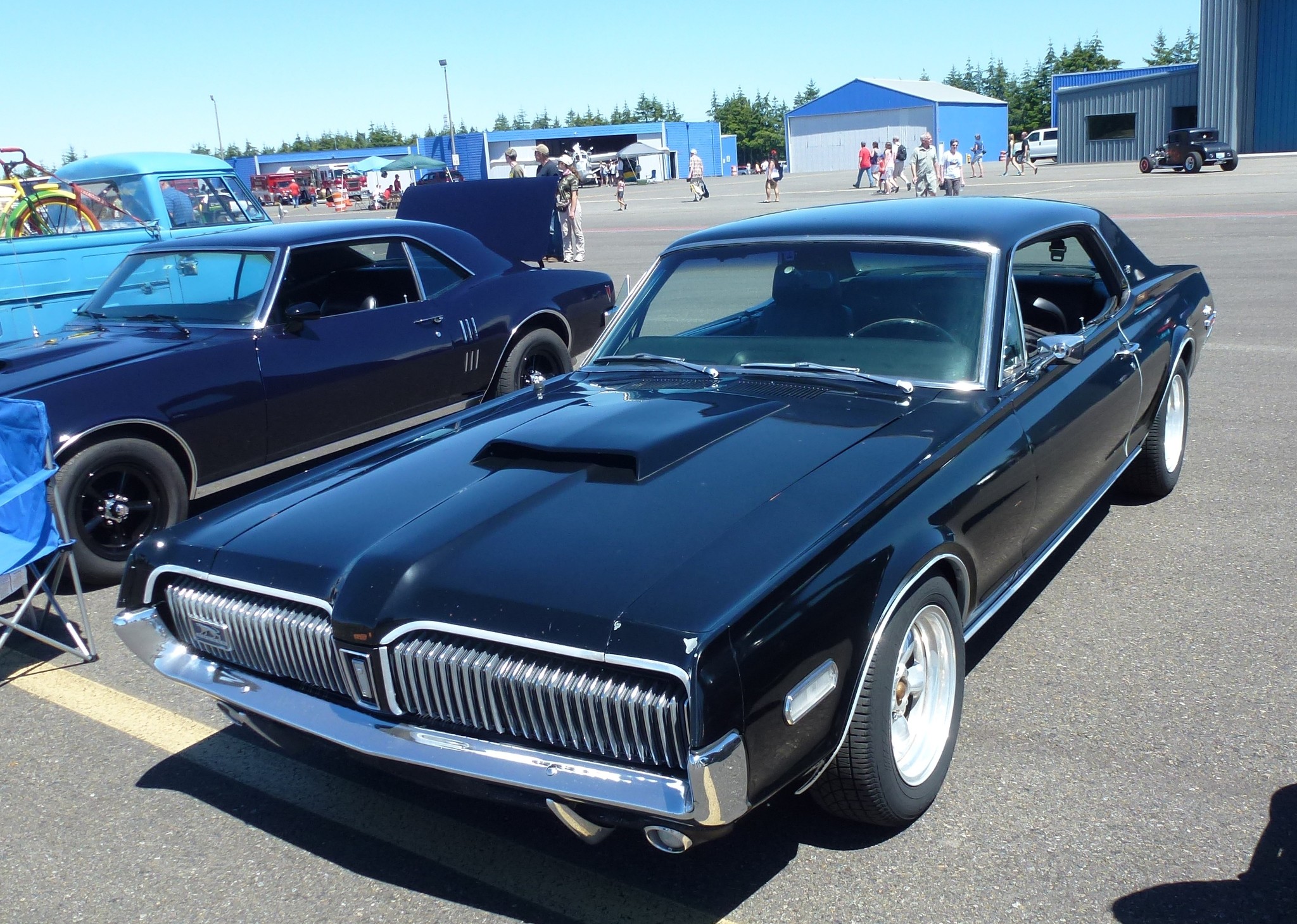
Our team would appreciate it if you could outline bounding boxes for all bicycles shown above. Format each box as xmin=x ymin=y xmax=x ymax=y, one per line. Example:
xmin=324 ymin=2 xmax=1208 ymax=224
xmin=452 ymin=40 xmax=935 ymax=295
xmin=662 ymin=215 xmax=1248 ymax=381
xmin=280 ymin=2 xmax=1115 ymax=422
xmin=0 ymin=147 xmax=104 ymax=239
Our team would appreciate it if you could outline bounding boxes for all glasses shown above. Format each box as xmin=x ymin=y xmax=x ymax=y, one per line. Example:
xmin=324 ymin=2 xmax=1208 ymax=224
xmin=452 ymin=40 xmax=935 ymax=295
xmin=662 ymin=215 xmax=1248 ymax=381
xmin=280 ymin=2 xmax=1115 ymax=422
xmin=952 ymin=144 xmax=958 ymax=146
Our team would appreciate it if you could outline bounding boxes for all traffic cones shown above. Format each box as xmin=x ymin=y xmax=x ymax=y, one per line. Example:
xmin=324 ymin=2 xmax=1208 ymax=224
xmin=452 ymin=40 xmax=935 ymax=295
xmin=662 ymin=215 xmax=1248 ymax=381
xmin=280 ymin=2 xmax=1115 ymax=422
xmin=345 ymin=193 xmax=352 ymax=207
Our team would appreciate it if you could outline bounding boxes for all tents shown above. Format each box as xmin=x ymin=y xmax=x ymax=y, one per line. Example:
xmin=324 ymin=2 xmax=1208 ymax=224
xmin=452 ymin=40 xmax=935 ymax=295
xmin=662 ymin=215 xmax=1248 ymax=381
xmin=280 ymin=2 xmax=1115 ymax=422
xmin=380 ymin=153 xmax=453 ymax=187
xmin=616 ymin=141 xmax=670 ymax=184
xmin=340 ymin=155 xmax=396 ymax=213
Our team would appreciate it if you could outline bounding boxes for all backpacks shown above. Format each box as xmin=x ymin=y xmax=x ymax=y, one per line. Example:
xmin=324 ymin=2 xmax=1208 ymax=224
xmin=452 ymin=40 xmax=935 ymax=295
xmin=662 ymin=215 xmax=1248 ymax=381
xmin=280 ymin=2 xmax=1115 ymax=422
xmin=774 ymin=165 xmax=784 ymax=182
xmin=895 ymin=144 xmax=906 ymax=160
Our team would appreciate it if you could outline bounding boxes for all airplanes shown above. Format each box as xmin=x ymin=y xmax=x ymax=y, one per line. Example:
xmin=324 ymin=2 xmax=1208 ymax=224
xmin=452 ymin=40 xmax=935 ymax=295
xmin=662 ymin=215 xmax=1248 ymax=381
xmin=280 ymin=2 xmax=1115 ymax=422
xmin=556 ymin=143 xmax=618 ymax=187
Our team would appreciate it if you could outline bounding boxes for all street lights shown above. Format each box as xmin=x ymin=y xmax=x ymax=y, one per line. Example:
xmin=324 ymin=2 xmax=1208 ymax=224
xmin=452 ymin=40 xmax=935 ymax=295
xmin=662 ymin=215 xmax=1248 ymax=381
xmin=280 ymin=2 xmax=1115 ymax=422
xmin=210 ymin=95 xmax=224 ymax=160
xmin=439 ymin=59 xmax=458 ymax=171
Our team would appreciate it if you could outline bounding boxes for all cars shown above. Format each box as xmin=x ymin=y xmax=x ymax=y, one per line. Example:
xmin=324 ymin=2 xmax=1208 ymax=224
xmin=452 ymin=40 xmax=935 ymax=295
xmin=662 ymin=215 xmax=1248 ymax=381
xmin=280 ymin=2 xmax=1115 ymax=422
xmin=111 ymin=199 xmax=1215 ymax=869
xmin=0 ymin=219 xmax=616 ymax=594
xmin=1139 ymin=127 xmax=1239 ymax=174
xmin=737 ymin=165 xmax=749 ymax=175
xmin=778 ymin=161 xmax=787 ymax=168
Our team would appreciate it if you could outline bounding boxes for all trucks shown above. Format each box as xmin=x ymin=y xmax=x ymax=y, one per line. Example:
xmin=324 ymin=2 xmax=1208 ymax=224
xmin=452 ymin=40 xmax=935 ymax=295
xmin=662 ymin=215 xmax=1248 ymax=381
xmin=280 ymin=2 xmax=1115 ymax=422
xmin=0 ymin=152 xmax=279 ymax=351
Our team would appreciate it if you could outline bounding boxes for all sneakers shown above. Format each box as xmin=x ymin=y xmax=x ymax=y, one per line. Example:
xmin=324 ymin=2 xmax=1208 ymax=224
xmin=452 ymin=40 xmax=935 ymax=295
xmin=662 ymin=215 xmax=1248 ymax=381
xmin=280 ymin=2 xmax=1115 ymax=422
xmin=563 ymin=253 xmax=574 ymax=263
xmin=548 ymin=257 xmax=562 ymax=262
xmin=574 ymin=253 xmax=585 ymax=261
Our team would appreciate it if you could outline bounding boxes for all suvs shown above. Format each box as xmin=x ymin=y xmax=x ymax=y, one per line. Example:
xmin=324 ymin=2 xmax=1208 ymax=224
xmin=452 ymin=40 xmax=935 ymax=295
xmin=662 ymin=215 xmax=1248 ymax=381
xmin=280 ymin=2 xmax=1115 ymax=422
xmin=1011 ymin=127 xmax=1058 ymax=163
xmin=410 ymin=169 xmax=465 ymax=188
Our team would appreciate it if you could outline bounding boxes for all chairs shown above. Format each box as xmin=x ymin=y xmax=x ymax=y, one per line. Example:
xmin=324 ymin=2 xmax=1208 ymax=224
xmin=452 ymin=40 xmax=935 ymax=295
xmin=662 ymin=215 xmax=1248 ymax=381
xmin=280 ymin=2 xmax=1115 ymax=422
xmin=378 ymin=193 xmax=401 ymax=210
xmin=757 ymin=262 xmax=844 ymax=337
xmin=354 ymin=196 xmax=367 ymax=211
xmin=0 ymin=397 xmax=97 ymax=661
xmin=645 ymin=170 xmax=657 ymax=184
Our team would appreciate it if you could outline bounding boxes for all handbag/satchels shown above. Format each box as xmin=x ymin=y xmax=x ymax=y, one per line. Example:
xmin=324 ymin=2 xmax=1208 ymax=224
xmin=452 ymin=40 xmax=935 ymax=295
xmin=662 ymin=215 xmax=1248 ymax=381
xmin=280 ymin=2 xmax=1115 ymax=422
xmin=700 ymin=179 xmax=709 ymax=198
xmin=879 ymin=161 xmax=885 ymax=174
xmin=870 ymin=148 xmax=877 ymax=164
xmin=938 ymin=180 xmax=948 ymax=190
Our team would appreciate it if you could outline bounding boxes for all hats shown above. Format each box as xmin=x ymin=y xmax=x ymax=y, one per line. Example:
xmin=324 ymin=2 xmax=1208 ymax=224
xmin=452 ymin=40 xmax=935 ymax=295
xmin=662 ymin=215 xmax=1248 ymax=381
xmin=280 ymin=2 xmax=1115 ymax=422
xmin=691 ymin=149 xmax=697 ymax=154
xmin=556 ymin=154 xmax=573 ymax=166
xmin=531 ymin=144 xmax=550 ymax=154
xmin=505 ymin=148 xmax=516 ymax=157
xmin=292 ymin=179 xmax=295 ymax=182
xmin=892 ymin=136 xmax=900 ymax=140
xmin=873 ymin=141 xmax=878 ymax=145
xmin=861 ymin=141 xmax=866 ymax=145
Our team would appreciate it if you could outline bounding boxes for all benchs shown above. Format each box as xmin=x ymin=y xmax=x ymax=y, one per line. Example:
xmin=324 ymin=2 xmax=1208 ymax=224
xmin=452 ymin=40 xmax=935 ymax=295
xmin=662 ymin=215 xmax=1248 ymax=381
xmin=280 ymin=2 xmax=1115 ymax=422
xmin=310 ymin=266 xmax=448 ymax=304
xmin=816 ymin=265 xmax=1105 ymax=336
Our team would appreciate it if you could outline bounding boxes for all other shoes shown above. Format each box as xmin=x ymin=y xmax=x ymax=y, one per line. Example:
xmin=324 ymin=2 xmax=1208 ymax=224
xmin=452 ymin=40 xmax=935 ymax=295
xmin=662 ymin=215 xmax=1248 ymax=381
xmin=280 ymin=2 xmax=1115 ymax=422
xmin=764 ymin=200 xmax=771 ymax=203
xmin=853 ymin=181 xmax=912 ymax=195
xmin=624 ymin=204 xmax=627 ymax=210
xmin=969 ymin=167 xmax=1038 ymax=178
xmin=618 ymin=208 xmax=623 ymax=211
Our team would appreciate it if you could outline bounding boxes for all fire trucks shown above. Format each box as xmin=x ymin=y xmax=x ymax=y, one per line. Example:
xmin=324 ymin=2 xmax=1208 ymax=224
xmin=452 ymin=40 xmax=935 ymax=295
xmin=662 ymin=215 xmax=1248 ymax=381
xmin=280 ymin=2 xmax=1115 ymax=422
xmin=249 ymin=163 xmax=368 ymax=207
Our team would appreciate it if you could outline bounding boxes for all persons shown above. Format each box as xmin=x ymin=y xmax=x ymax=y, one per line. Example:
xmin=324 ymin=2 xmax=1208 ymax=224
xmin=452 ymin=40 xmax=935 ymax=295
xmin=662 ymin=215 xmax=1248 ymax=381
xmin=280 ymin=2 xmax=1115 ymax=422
xmin=614 ymin=176 xmax=627 ymax=211
xmin=1019 ymin=131 xmax=1038 ymax=176
xmin=394 ymin=174 xmax=403 ymax=196
xmin=308 ymin=182 xmax=318 ymax=203
xmin=533 ymin=143 xmax=563 ymax=262
xmin=598 ymin=157 xmax=625 ymax=188
xmin=160 ymin=180 xmax=197 ymax=225
xmin=853 ymin=136 xmax=911 ymax=195
xmin=1000 ymin=134 xmax=1022 ymax=176
xmin=372 ymin=184 xmax=383 ymax=202
xmin=763 ymin=149 xmax=779 ymax=202
xmin=939 ymin=139 xmax=965 ymax=196
xmin=688 ymin=149 xmax=705 ymax=202
xmin=745 ymin=159 xmax=769 ymax=175
xmin=556 ymin=154 xmax=586 ymax=262
xmin=288 ymin=179 xmax=301 ymax=208
xmin=383 ymin=185 xmax=400 ymax=208
xmin=909 ymin=133 xmax=941 ymax=197
xmin=970 ymin=134 xmax=985 ymax=177
xmin=504 ymin=148 xmax=524 ymax=179
